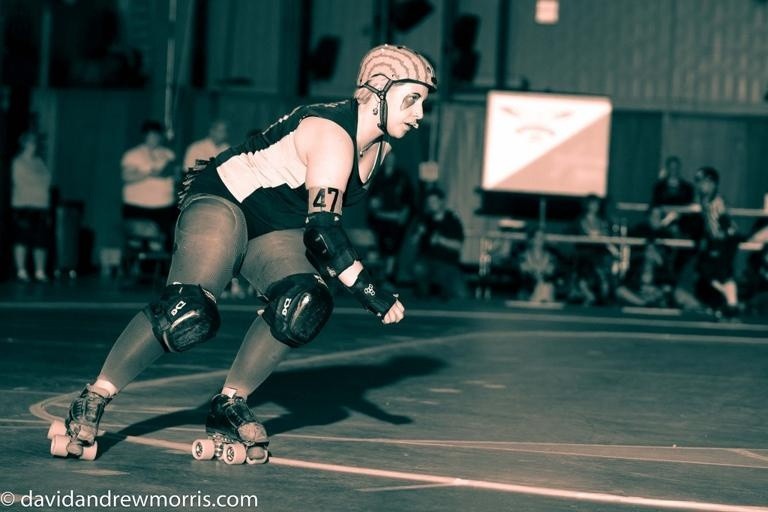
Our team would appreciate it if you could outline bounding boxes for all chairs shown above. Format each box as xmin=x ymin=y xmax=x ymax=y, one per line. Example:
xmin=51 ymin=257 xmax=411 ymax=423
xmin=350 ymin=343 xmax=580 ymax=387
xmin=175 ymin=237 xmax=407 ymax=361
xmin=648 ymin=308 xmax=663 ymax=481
xmin=344 ymin=226 xmax=387 ymax=290
xmin=113 ymin=221 xmax=171 ymax=292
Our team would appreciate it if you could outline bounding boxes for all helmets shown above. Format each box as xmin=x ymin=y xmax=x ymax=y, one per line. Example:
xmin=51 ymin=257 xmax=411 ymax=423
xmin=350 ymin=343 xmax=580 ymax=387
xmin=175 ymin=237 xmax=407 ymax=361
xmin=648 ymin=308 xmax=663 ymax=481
xmin=356 ymin=43 xmax=437 ymax=91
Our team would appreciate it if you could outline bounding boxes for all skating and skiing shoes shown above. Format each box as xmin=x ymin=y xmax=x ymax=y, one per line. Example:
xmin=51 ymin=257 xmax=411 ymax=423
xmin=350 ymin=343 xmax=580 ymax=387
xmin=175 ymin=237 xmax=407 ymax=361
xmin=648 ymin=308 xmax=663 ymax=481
xmin=47 ymin=384 xmax=106 ymax=460
xmin=192 ymin=393 xmax=269 ymax=465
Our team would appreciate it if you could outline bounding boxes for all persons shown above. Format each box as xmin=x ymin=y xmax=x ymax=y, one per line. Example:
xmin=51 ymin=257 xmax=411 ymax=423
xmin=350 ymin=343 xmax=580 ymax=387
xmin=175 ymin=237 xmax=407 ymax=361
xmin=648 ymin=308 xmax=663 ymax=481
xmin=118 ymin=120 xmax=174 ymax=286
xmin=10 ymin=131 xmax=52 ymax=284
xmin=65 ymin=43 xmax=439 ymax=446
xmin=410 ymin=189 xmax=464 ymax=302
xmin=366 ymin=152 xmax=415 ymax=279
xmin=646 ymin=155 xmax=695 ymax=284
xmin=183 ymin=118 xmax=232 ymax=169
xmin=575 ymin=194 xmax=616 ymax=309
xmin=694 ymin=167 xmax=744 ymax=317
